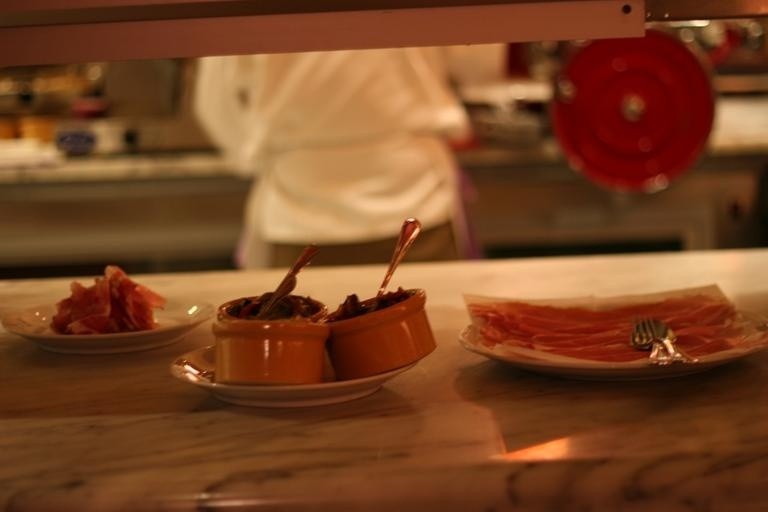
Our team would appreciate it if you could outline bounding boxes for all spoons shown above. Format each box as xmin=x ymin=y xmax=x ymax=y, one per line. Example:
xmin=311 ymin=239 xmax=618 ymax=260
xmin=632 ymin=318 xmax=678 ymax=368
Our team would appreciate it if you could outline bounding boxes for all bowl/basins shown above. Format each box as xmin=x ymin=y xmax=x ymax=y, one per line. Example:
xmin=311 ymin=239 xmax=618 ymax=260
xmin=211 ymin=287 xmax=438 ymax=387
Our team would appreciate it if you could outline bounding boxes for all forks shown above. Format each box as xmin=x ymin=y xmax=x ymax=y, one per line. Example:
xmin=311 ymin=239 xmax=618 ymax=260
xmin=630 ymin=313 xmax=700 ymax=368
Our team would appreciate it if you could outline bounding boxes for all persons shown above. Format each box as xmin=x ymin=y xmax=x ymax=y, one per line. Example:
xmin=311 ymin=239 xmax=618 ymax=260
xmin=190 ymin=47 xmax=474 ymax=269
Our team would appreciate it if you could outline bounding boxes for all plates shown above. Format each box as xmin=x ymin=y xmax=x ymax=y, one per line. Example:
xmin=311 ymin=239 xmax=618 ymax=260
xmin=168 ymin=342 xmax=419 ymax=409
xmin=2 ymin=293 xmax=217 ymax=355
xmin=457 ymin=279 xmax=768 ymax=382
